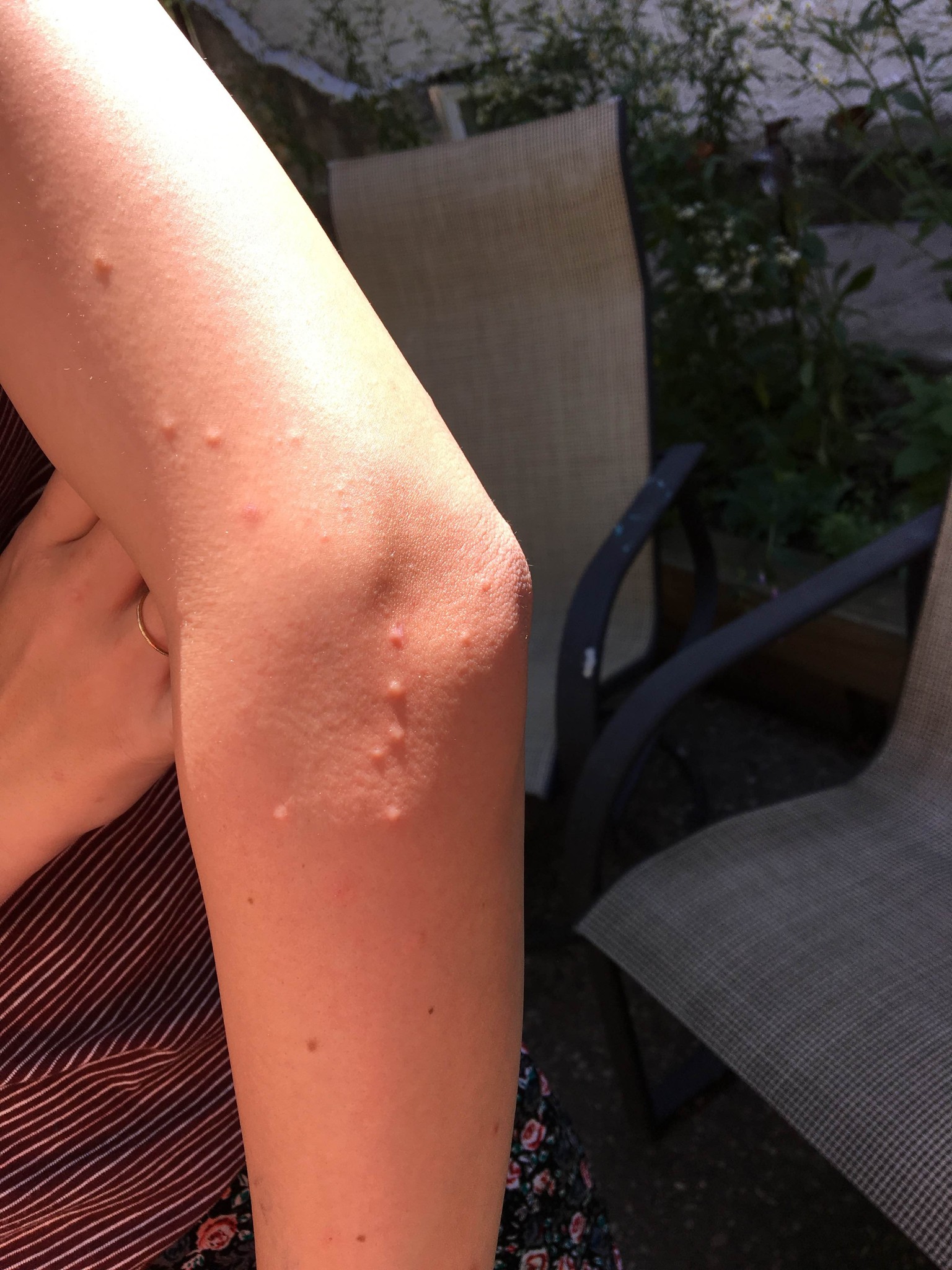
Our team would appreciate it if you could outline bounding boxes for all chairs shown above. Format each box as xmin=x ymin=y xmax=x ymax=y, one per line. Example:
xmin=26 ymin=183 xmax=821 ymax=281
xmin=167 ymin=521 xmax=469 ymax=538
xmin=584 ymin=472 xmax=952 ymax=1270
xmin=325 ymin=90 xmax=729 ymax=874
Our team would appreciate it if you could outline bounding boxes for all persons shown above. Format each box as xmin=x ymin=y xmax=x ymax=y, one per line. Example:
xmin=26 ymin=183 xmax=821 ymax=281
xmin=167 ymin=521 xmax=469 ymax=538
xmin=2 ymin=0 xmax=628 ymax=1270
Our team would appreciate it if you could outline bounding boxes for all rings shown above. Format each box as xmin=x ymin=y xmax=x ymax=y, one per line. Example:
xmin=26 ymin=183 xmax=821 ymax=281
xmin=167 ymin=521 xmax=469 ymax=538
xmin=136 ymin=593 xmax=171 ymax=660
